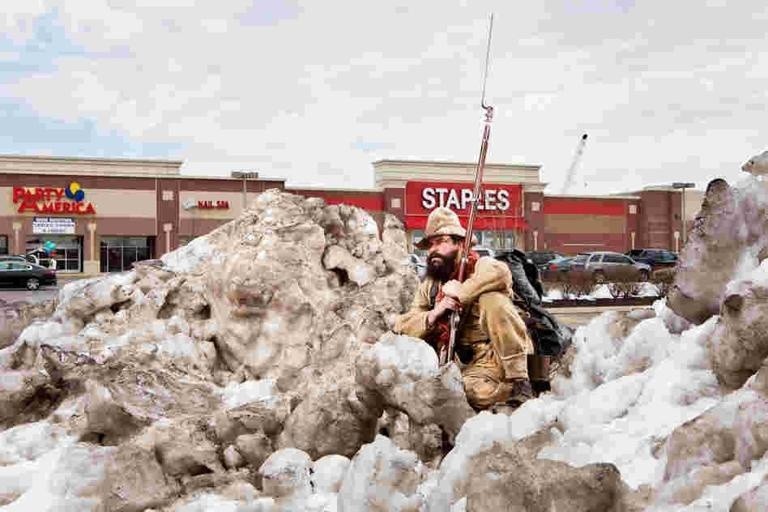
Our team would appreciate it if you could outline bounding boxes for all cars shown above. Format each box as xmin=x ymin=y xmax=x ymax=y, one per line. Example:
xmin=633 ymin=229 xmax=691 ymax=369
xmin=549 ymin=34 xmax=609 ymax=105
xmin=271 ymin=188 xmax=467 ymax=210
xmin=407 ymin=254 xmax=428 ymax=283
xmin=0 ymin=247 xmax=59 ymax=292
xmin=476 ymin=247 xmax=683 ymax=285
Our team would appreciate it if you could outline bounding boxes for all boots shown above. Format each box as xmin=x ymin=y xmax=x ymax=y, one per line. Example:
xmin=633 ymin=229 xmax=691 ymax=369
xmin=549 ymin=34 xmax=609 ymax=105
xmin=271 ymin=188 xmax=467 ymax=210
xmin=505 ymin=379 xmax=533 ymax=407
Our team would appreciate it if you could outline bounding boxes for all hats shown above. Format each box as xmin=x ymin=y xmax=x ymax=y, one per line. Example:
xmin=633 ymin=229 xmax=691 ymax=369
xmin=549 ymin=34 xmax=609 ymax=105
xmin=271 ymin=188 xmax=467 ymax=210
xmin=415 ymin=206 xmax=479 ymax=251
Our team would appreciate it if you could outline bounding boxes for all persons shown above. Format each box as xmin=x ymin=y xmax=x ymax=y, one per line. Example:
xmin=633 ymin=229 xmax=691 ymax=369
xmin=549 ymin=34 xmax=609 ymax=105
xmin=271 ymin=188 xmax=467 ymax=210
xmin=392 ymin=204 xmax=535 ymax=410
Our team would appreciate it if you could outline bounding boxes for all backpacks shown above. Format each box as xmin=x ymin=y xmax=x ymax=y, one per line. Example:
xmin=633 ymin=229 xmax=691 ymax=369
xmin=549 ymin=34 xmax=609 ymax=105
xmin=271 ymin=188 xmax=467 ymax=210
xmin=490 ymin=249 xmax=562 ymax=355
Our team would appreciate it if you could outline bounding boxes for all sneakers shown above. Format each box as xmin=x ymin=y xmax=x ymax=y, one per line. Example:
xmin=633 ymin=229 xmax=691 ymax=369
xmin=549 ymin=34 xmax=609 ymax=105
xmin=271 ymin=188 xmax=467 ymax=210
xmin=530 ymin=378 xmax=551 ymax=398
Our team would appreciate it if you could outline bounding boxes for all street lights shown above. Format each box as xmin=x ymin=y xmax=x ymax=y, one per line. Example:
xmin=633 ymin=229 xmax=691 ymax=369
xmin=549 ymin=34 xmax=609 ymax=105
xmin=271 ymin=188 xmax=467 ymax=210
xmin=672 ymin=182 xmax=695 ymax=247
xmin=230 ymin=171 xmax=261 ymax=214
